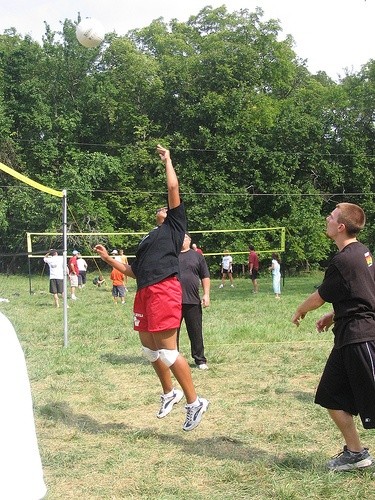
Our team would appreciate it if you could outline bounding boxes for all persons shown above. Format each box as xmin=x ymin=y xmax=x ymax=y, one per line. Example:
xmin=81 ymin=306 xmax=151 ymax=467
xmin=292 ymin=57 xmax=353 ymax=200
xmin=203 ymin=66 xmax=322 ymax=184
xmin=291 ymin=200 xmax=375 ymax=471
xmin=92 ymin=275 xmax=106 ymax=290
xmin=92 ymin=143 xmax=208 ymax=433
xmin=192 ymin=242 xmax=204 ymax=255
xmin=43 ymin=248 xmax=70 ymax=309
xmin=217 ymin=248 xmax=237 ymax=289
xmin=0 ymin=296 xmax=49 ymax=500
xmin=77 ymin=254 xmax=88 ymax=289
xmin=177 ymin=232 xmax=210 ymax=371
xmin=67 ymin=249 xmax=80 ymax=301
xmin=246 ymin=244 xmax=262 ymax=295
xmin=267 ymin=251 xmax=286 ymax=299
xmin=108 ymin=247 xmax=129 ymax=305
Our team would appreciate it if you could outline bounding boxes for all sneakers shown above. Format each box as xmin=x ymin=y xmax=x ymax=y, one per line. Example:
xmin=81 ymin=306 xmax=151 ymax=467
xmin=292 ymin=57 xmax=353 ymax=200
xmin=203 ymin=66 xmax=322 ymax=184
xmin=182 ymin=397 xmax=208 ymax=431
xmin=327 ymin=445 xmax=372 ymax=471
xmin=156 ymin=388 xmax=184 ymax=419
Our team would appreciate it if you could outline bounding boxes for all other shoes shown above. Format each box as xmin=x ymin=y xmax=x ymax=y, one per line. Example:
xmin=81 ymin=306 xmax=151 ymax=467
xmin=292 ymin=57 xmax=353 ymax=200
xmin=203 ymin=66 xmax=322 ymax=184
xmin=196 ymin=364 xmax=209 ymax=370
xmin=230 ymin=285 xmax=234 ymax=288
xmin=219 ymin=284 xmax=223 ymax=289
xmin=70 ymin=296 xmax=80 ymax=301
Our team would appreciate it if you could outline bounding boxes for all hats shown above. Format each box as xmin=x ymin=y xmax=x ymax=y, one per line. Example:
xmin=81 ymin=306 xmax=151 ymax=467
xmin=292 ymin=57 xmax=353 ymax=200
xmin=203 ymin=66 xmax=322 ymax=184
xmin=111 ymin=250 xmax=118 ymax=255
xmin=72 ymin=250 xmax=80 ymax=255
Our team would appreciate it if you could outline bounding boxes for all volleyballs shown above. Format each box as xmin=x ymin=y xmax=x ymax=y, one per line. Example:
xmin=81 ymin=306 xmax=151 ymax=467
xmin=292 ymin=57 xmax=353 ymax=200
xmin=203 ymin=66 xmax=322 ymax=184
xmin=74 ymin=16 xmax=104 ymax=48
